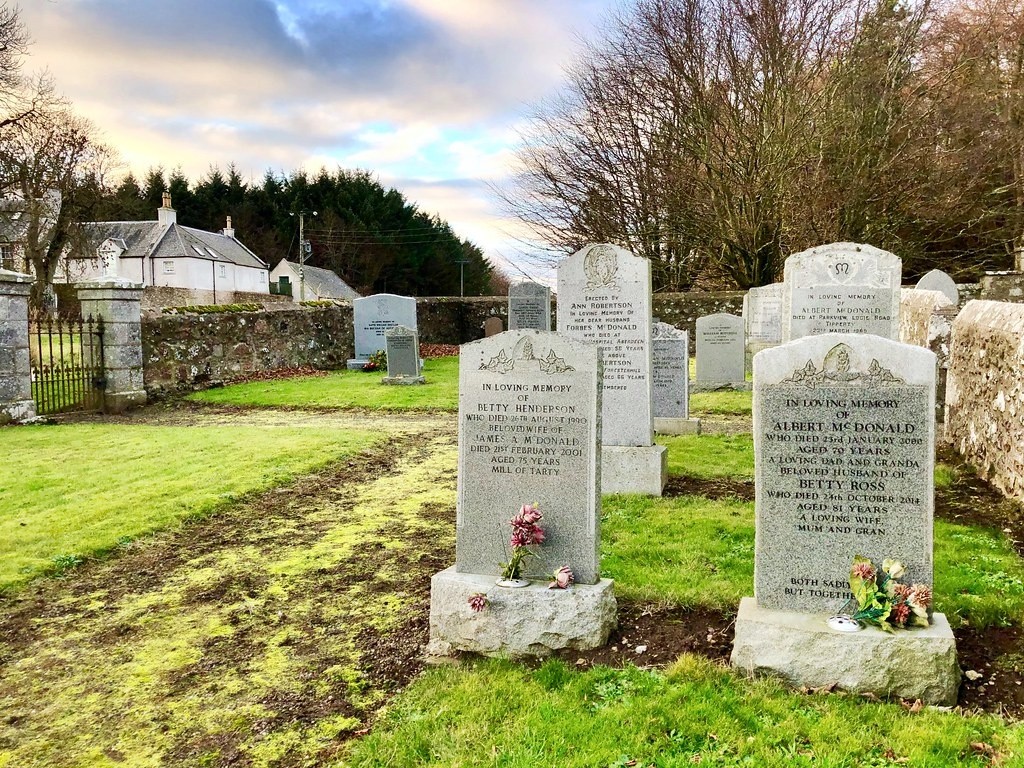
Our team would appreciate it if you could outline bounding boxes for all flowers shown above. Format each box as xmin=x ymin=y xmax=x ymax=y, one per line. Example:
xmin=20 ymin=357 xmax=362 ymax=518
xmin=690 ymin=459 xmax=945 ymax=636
xmin=468 ymin=500 xmax=578 ymax=612
xmin=838 ymin=554 xmax=936 ymax=634
xmin=364 ymin=349 xmax=388 ymax=372
